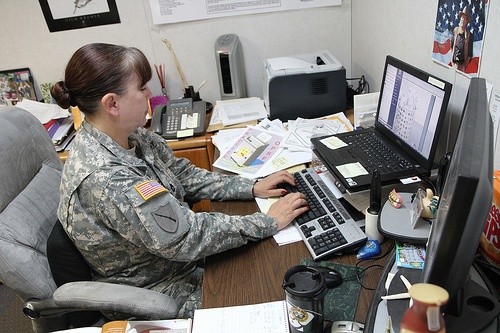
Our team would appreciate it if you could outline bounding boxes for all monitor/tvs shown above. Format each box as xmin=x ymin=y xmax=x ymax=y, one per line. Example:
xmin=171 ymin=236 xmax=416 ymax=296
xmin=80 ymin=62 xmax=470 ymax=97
xmin=387 ymin=77 xmax=500 ymax=333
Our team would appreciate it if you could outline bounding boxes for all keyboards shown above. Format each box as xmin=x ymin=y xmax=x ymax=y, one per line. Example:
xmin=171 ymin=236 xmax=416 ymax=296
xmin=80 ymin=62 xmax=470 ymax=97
xmin=273 ymin=168 xmax=367 ymax=261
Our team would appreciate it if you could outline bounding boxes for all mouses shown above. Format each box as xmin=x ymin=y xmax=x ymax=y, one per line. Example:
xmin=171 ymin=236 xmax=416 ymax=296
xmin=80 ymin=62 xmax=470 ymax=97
xmin=309 ymin=266 xmax=343 ymax=287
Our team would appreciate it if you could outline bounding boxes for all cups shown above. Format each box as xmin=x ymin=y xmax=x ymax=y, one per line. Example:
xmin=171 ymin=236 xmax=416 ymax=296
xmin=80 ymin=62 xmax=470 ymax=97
xmin=282 ymin=265 xmax=328 ymax=333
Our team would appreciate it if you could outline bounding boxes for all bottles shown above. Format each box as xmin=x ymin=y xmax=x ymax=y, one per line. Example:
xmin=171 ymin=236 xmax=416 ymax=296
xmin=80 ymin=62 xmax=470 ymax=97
xmin=399 ymin=283 xmax=449 ymax=333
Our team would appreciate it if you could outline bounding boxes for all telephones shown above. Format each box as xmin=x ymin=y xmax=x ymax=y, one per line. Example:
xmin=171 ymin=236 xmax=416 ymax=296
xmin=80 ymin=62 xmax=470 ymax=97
xmin=151 ymin=98 xmax=207 ymax=139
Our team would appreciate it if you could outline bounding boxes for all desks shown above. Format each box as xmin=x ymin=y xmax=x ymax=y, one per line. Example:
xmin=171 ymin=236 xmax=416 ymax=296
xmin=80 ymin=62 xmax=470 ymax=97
xmin=191 ymin=145 xmax=500 ymax=333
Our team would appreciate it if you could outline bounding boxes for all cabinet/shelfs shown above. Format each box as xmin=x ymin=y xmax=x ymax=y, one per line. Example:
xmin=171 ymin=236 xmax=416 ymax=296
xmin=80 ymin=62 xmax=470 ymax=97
xmin=56 ymin=105 xmax=355 ymax=213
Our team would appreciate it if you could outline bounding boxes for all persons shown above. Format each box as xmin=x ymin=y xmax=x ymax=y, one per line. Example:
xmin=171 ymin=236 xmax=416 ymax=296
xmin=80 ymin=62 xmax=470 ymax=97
xmin=50 ymin=42 xmax=311 ymax=321
xmin=450 ymin=6 xmax=473 ymax=73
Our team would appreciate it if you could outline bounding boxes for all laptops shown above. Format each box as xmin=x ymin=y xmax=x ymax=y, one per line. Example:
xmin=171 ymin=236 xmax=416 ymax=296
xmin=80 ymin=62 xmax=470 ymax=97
xmin=310 ymin=55 xmax=453 ymax=193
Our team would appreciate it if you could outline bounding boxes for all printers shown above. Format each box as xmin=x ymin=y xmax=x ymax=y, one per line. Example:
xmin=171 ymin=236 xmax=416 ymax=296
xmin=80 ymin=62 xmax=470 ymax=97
xmin=263 ymin=50 xmax=348 ymax=122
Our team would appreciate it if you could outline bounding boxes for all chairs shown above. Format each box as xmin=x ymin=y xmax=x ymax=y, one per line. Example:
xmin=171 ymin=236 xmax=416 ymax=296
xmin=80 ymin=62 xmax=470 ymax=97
xmin=0 ymin=104 xmax=178 ymax=333
